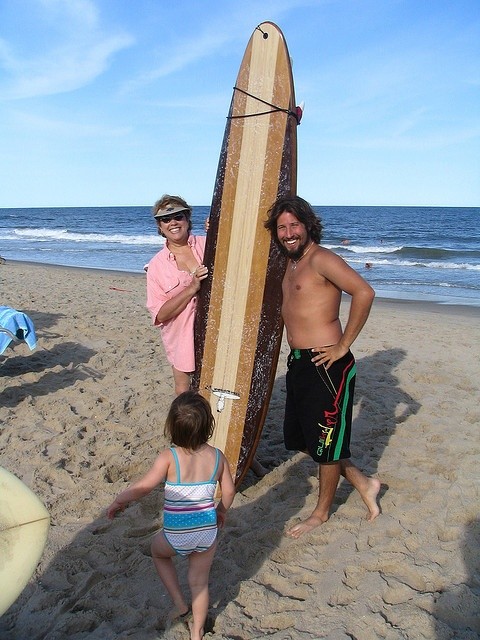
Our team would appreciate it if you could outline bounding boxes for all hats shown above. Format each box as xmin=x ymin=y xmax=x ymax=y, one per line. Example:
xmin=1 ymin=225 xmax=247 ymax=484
xmin=153 ymin=199 xmax=191 ymax=217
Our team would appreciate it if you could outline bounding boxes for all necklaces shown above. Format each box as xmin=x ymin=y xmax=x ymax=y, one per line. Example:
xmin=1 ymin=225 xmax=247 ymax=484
xmin=290 ymin=241 xmax=315 ymax=272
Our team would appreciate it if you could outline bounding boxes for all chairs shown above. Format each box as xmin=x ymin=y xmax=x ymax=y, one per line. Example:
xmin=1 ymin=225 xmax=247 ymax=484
xmin=0 ymin=309 xmax=36 ymax=358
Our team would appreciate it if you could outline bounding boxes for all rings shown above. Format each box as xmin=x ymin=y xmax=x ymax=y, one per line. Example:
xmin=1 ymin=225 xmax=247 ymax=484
xmin=195 ymin=275 xmax=198 ymax=277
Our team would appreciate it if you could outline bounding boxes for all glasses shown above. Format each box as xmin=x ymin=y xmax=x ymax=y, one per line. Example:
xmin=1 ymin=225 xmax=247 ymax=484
xmin=158 ymin=212 xmax=184 ymax=223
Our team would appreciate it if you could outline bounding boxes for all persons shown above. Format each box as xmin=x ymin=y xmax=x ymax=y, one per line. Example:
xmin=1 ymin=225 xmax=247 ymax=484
xmin=107 ymin=392 xmax=235 ymax=639
xmin=144 ymin=194 xmax=209 ymax=393
xmin=266 ymin=198 xmax=381 ymax=538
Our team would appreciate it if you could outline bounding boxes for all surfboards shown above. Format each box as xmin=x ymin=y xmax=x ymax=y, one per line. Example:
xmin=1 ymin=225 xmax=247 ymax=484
xmin=190 ymin=21 xmax=306 ymax=493
